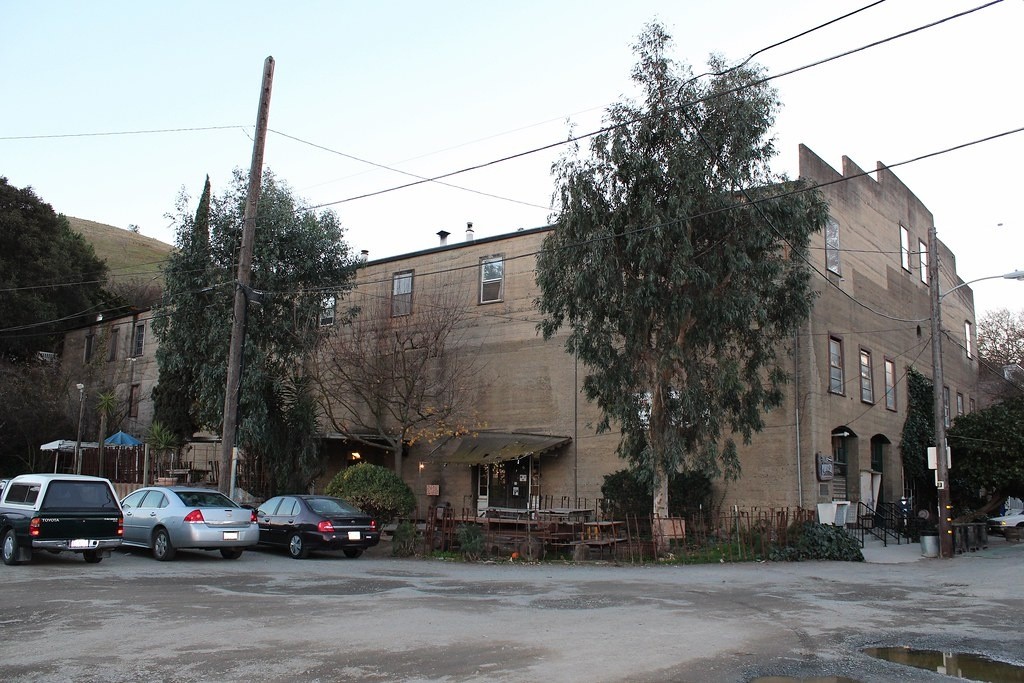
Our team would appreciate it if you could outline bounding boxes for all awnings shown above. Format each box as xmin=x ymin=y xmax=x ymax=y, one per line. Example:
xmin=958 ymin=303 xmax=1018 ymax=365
xmin=422 ymin=430 xmax=573 ymax=464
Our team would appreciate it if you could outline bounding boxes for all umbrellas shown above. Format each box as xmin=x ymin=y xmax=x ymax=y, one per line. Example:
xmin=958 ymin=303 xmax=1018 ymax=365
xmin=96 ymin=429 xmax=144 ymax=465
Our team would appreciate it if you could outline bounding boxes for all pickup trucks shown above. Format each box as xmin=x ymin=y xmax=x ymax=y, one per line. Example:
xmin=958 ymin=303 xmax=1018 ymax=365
xmin=0 ymin=473 xmax=125 ymax=564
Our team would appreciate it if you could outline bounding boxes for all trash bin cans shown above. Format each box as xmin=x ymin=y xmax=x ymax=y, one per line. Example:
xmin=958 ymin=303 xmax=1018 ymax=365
xmin=920 ymin=529 xmax=940 ymax=556
xmin=952 ymin=522 xmax=988 ymax=554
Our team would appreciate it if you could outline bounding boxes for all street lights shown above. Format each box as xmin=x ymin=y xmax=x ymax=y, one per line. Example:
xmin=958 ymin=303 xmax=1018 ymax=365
xmin=73 ymin=383 xmax=87 ymax=475
xmin=929 ymin=269 xmax=1024 ymax=546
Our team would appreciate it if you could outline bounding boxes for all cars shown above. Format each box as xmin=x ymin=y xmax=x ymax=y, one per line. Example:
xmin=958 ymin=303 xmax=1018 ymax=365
xmin=117 ymin=485 xmax=260 ymax=561
xmin=250 ymin=495 xmax=380 ymax=560
xmin=986 ymin=508 xmax=1024 ymax=537
